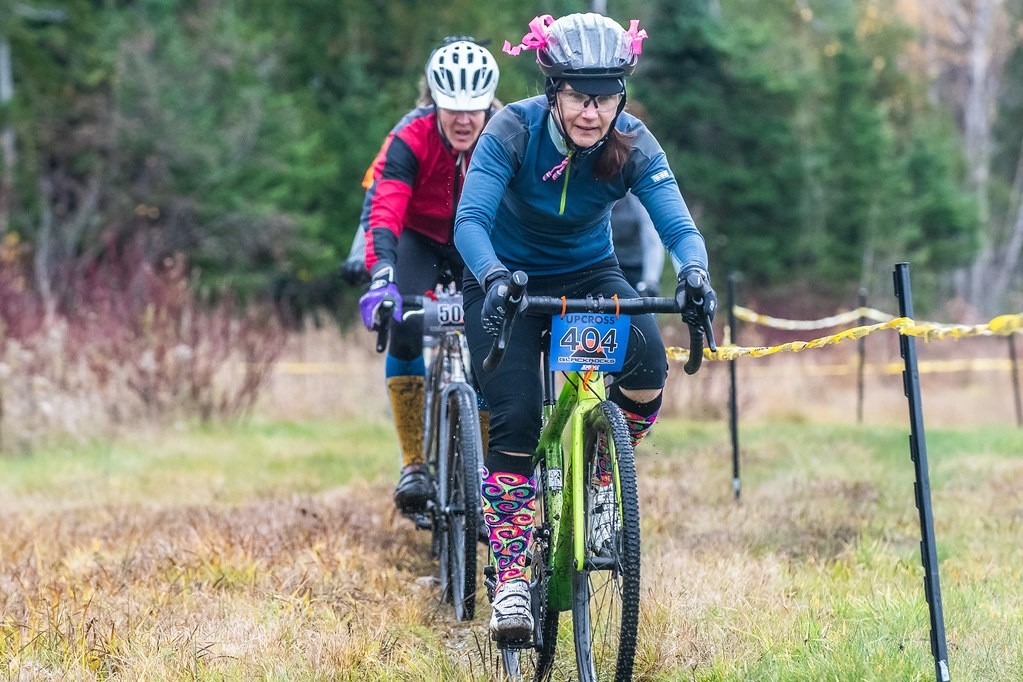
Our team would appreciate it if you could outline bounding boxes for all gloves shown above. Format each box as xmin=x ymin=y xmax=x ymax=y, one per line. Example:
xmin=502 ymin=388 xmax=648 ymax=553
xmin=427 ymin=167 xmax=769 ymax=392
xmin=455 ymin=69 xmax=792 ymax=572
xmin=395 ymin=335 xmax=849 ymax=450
xmin=358 ymin=280 xmax=403 ymax=332
xmin=480 ymin=273 xmax=529 ymax=336
xmin=675 ymin=266 xmax=716 ymax=334
xmin=342 ymin=259 xmax=372 ymax=288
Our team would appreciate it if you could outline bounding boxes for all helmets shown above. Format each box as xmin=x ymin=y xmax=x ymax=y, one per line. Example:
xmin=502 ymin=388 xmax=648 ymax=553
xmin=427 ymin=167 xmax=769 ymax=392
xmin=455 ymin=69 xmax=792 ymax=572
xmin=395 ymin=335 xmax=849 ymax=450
xmin=425 ymin=35 xmax=479 ymax=71
xmin=536 ymin=14 xmax=639 ymax=79
xmin=427 ymin=40 xmax=500 ymax=110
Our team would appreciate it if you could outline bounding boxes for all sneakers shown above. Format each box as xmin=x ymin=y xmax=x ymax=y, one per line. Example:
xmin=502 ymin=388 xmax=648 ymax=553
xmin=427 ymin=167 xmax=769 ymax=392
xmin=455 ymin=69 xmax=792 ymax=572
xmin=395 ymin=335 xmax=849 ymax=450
xmin=394 ymin=463 xmax=438 ymax=510
xmin=586 ymin=483 xmax=623 ymax=558
xmin=489 ymin=580 xmax=534 ymax=641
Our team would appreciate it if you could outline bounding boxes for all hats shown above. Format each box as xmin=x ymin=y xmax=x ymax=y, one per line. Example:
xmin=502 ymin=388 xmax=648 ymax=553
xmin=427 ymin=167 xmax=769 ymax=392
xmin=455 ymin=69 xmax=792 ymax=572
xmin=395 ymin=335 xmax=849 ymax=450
xmin=564 ymin=75 xmax=623 ymax=96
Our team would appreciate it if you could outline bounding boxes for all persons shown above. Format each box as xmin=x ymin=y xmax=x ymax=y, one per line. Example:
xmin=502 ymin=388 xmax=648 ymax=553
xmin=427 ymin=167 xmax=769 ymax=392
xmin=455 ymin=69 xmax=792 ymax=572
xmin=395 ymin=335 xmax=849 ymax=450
xmin=343 ymin=72 xmax=436 ymax=287
xmin=359 ymin=43 xmax=505 ymax=543
xmin=451 ymin=10 xmax=719 ymax=641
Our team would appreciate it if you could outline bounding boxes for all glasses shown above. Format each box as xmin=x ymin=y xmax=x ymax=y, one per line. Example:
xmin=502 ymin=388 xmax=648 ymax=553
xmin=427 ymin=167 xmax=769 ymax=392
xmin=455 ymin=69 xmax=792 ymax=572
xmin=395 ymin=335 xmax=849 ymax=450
xmin=557 ymin=87 xmax=626 ymax=113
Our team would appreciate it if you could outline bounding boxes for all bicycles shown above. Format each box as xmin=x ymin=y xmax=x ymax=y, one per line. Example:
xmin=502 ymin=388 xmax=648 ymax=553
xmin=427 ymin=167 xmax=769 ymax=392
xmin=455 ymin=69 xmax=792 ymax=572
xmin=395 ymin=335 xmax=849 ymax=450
xmin=477 ymin=267 xmax=720 ymax=682
xmin=374 ymin=284 xmax=484 ymax=625
